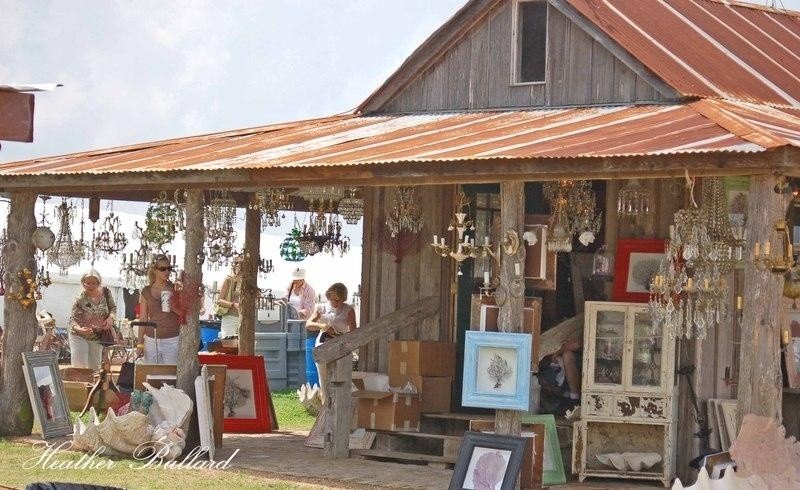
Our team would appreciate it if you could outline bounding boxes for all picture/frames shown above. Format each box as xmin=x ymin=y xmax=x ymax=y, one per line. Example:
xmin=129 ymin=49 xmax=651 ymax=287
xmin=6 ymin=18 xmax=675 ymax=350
xmin=450 ymin=414 xmax=567 ymax=489
xmin=463 ymin=328 xmax=533 ymax=410
xmin=612 ymin=237 xmax=687 ymax=301
xmin=21 ymin=351 xmax=74 ymax=440
xmin=201 ymin=356 xmax=272 ymax=433
xmin=305 ymin=407 xmax=376 ymax=452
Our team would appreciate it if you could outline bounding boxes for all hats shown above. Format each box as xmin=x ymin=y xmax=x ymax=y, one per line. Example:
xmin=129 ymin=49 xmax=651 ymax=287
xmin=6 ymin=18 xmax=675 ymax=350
xmin=292 ymin=268 xmax=304 ymax=280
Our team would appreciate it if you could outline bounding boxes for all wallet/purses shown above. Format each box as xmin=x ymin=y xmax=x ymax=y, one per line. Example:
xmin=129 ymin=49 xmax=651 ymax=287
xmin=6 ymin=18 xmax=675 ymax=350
xmin=319 ymin=332 xmax=342 ymax=342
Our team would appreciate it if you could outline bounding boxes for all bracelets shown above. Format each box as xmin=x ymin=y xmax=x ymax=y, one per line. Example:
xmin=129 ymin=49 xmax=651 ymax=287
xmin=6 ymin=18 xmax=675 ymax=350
xmin=137 ymin=341 xmax=144 ymax=349
xmin=231 ymin=302 xmax=235 ymax=308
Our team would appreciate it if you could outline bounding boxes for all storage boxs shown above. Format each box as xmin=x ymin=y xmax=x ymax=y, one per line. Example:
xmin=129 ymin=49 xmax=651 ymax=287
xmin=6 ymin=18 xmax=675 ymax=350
xmin=350 ymin=340 xmax=455 ymax=433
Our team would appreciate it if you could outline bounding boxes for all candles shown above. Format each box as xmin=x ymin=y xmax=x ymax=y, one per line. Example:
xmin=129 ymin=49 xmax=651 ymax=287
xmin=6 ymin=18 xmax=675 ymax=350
xmin=784 ymin=330 xmax=790 ymax=343
xmin=432 ymin=230 xmax=522 ymax=286
xmin=651 ymin=275 xmax=730 ymax=290
xmin=752 ymin=240 xmax=795 ymax=260
xmin=737 ymin=297 xmax=743 ymax=310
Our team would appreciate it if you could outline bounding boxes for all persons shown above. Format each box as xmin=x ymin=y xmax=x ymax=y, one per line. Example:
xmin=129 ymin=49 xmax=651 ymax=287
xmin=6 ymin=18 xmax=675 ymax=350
xmin=538 ymin=347 xmax=585 ymax=417
xmin=214 ymin=254 xmax=247 ymax=339
xmin=197 ymin=286 xmax=206 ymax=351
xmin=135 ymin=255 xmax=185 ymax=366
xmin=68 ymin=270 xmax=118 ymax=373
xmin=284 ymin=268 xmax=316 ymax=319
xmin=306 ymin=281 xmax=359 ymax=405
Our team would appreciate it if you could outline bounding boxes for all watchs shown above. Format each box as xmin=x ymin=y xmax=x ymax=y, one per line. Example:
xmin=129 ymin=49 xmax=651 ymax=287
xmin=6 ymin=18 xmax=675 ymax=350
xmin=108 ymin=316 xmax=116 ymax=322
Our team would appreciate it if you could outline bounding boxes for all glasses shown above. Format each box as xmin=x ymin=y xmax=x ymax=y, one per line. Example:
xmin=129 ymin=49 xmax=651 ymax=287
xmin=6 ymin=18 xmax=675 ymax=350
xmin=157 ymin=266 xmax=172 ymax=271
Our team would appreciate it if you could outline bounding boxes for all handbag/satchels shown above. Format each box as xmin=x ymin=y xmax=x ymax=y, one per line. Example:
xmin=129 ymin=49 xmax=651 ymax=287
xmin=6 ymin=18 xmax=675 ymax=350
xmin=214 ymin=288 xmax=232 ymax=315
xmin=101 ymin=327 xmax=123 ymax=345
xmin=117 ymin=362 xmax=134 ymax=388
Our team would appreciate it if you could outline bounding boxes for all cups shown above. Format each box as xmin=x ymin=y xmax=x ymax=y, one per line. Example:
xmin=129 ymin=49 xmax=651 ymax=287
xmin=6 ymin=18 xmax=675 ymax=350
xmin=161 ymin=290 xmax=171 ymax=312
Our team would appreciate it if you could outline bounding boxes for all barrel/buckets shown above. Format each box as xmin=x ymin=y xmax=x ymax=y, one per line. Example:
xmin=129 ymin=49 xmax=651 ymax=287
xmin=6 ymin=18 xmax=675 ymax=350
xmin=199 ymin=319 xmax=221 ymax=351
xmin=306 ymin=330 xmax=319 ymax=389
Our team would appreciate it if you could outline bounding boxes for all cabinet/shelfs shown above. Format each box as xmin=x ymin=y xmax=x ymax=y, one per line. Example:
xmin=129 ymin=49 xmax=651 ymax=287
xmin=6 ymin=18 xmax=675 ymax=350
xmin=572 ymin=302 xmax=681 ymax=489
